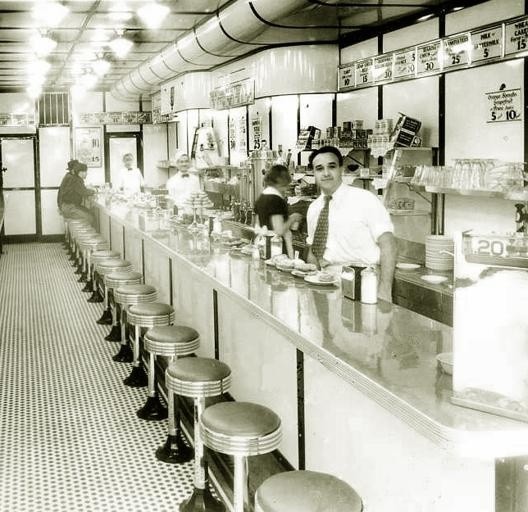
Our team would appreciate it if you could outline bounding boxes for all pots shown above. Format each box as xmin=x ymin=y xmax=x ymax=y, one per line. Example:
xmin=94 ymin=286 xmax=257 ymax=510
xmin=299 ymin=178 xmax=317 ymax=194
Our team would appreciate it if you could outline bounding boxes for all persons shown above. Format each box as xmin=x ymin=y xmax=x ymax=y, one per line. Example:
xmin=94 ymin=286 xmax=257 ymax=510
xmin=56 ymin=159 xmax=89 ymax=213
xmin=305 ymin=146 xmax=399 ymax=303
xmin=298 ymin=276 xmax=392 ymax=374
xmin=112 ymin=152 xmax=150 ymax=197
xmin=252 ymin=164 xmax=303 ymax=263
xmin=161 ymin=152 xmax=205 ymax=222
xmin=61 ymin=163 xmax=98 ymax=230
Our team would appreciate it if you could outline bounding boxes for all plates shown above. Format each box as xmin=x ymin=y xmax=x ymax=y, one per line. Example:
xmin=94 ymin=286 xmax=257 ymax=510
xmin=394 ymin=261 xmax=420 ymax=271
xmin=264 ymin=259 xmax=338 ymax=286
xmin=420 ymin=274 xmax=448 ymax=285
xmin=423 ymin=234 xmax=453 ymax=272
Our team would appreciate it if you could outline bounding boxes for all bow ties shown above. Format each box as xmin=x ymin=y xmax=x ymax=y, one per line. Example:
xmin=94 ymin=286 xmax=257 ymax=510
xmin=128 ymin=168 xmax=132 ymax=170
xmin=181 ymin=173 xmax=189 ymax=177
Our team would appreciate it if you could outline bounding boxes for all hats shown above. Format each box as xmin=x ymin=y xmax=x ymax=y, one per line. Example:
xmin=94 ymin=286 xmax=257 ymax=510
xmin=65 ymin=159 xmax=78 ymax=170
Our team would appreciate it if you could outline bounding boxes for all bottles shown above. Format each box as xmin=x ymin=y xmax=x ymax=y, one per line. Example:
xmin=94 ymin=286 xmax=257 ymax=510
xmin=359 ymin=264 xmax=378 ymax=304
xmin=270 ymin=234 xmax=282 ymax=258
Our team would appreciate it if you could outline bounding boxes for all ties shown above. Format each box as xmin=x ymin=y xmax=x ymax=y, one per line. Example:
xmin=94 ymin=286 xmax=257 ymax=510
xmin=311 ymin=195 xmax=332 ymax=271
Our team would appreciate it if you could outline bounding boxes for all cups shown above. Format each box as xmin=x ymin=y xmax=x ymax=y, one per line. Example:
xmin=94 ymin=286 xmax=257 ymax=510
xmin=409 ymin=156 xmax=496 ymax=190
